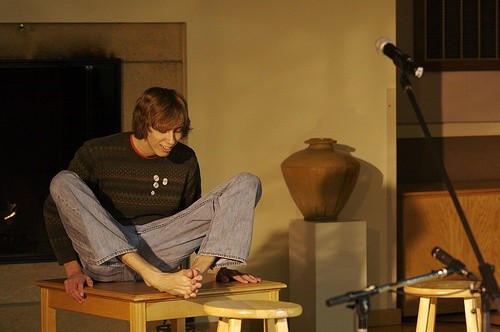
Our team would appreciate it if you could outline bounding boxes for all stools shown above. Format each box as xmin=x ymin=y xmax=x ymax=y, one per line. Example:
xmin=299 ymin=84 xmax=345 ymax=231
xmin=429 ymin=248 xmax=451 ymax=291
xmin=404 ymin=279 xmax=488 ymax=332
xmin=203 ymin=300 xmax=302 ymax=332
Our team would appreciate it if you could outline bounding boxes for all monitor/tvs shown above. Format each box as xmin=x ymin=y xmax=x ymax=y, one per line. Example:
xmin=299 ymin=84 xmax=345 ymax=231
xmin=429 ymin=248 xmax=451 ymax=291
xmin=0 ymin=57 xmax=122 ymax=264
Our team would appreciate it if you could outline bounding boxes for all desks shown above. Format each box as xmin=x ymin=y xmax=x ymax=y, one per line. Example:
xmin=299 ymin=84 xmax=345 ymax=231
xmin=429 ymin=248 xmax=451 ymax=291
xmin=398 ymin=181 xmax=500 ymax=316
xmin=289 ymin=218 xmax=367 ymax=331
xmin=32 ymin=273 xmax=286 ymax=332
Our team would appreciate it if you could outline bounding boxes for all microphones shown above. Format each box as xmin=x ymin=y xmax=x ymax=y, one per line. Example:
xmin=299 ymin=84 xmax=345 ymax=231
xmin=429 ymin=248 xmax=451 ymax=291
xmin=375 ymin=36 xmax=424 ymax=78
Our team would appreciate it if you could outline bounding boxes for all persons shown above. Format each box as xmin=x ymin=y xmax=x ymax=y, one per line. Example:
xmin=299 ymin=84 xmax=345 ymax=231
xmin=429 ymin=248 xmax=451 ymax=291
xmin=43 ymin=87 xmax=262 ymax=304
